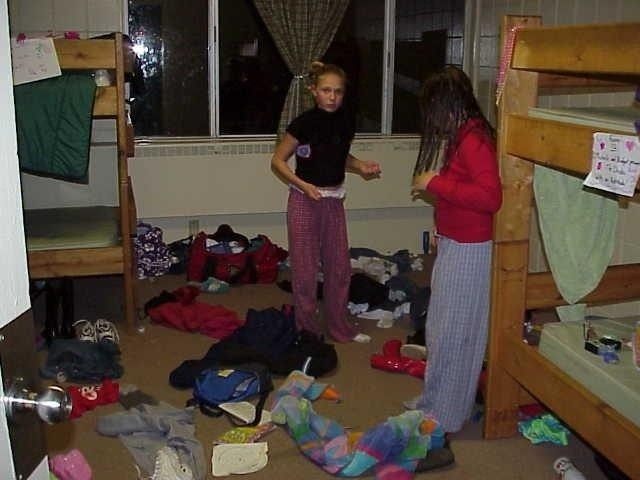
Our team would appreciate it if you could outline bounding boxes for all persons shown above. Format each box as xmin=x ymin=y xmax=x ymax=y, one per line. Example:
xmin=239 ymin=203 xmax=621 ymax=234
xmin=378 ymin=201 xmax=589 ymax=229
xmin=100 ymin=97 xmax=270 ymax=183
xmin=404 ymin=67 xmax=503 ymax=434
xmin=271 ymin=60 xmax=382 ymax=343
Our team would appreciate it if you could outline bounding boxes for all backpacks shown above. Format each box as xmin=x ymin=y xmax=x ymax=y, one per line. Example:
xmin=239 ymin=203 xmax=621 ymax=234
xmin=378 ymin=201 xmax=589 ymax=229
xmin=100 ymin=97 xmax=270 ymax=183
xmin=194 ymin=362 xmax=274 ymax=426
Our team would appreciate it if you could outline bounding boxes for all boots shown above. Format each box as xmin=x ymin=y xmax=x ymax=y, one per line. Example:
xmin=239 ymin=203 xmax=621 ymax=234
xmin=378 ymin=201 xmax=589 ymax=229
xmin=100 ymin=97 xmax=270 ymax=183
xmin=400 ymin=329 xmax=428 ymax=359
xmin=371 ymin=340 xmax=426 ymax=377
xmin=61 ymin=278 xmax=74 ymax=339
xmin=40 ymin=283 xmax=59 ymax=343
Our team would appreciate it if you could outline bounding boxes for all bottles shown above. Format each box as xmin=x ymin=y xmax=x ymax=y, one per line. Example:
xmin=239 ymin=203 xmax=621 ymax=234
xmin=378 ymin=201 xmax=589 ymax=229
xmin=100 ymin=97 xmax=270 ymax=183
xmin=94 ymin=69 xmax=113 ymax=86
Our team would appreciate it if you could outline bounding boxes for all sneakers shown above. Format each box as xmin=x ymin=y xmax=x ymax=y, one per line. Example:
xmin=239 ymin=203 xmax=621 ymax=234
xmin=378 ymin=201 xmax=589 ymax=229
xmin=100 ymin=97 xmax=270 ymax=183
xmin=72 ymin=319 xmax=119 ymax=345
xmin=416 ymin=449 xmax=453 ymax=471
xmin=149 ymin=448 xmax=192 ymax=480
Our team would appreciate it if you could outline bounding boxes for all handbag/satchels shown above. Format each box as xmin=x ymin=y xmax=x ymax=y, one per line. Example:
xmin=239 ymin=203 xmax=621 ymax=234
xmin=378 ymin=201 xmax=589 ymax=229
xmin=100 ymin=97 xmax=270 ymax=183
xmin=129 ymin=222 xmax=288 ymax=286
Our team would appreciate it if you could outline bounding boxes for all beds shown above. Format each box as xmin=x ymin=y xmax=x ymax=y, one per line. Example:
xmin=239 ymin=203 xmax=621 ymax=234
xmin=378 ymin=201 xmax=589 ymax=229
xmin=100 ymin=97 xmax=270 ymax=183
xmin=485 ymin=21 xmax=640 ymax=480
xmin=9 ymin=31 xmax=141 ymax=341
